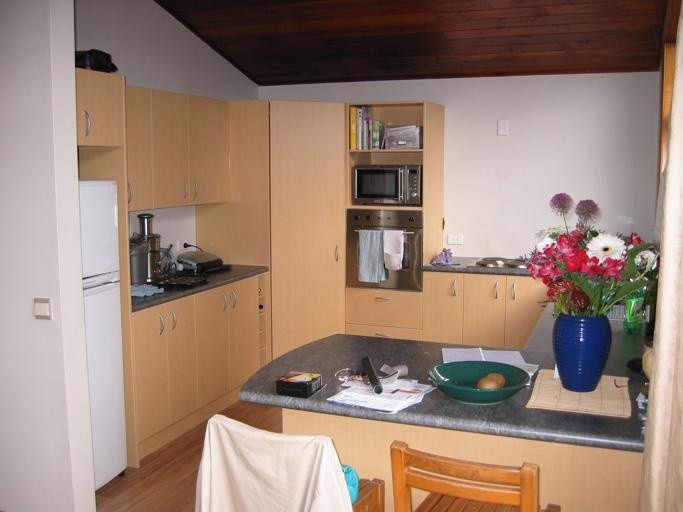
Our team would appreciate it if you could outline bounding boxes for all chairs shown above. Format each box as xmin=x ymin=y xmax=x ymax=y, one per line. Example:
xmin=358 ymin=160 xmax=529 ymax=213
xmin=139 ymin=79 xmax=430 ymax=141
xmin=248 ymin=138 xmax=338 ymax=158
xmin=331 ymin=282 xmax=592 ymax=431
xmin=388 ymin=444 xmax=560 ymax=512
xmin=196 ymin=406 xmax=381 ymax=512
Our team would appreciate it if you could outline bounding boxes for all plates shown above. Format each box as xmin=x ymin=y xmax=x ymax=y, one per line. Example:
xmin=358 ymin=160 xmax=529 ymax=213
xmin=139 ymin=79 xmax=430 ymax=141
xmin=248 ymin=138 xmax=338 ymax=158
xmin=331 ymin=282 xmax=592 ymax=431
xmin=152 ymin=273 xmax=207 ymax=287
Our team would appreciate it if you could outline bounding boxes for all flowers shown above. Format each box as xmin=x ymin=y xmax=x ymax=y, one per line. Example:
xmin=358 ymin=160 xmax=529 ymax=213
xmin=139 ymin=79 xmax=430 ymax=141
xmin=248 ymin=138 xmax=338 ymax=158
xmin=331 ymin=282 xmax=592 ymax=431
xmin=527 ymin=193 xmax=659 ymax=316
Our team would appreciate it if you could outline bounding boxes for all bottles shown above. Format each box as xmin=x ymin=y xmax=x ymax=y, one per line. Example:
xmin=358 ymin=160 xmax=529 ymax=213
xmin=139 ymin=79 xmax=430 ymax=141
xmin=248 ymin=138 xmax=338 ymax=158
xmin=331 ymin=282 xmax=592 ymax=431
xmin=642 ymin=341 xmax=652 ymax=379
xmin=129 ymin=213 xmax=173 ymax=286
xmin=623 ymin=278 xmax=658 ymax=340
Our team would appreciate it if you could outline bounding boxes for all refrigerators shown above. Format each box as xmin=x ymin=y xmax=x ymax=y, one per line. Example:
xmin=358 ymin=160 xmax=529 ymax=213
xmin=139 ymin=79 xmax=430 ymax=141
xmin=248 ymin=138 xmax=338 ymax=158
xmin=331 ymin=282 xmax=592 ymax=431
xmin=73 ymin=180 xmax=129 ymax=494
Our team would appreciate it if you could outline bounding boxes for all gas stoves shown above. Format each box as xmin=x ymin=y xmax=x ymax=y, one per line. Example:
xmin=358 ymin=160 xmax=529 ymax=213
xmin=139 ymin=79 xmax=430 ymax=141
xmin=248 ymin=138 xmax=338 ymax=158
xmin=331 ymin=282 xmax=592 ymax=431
xmin=466 ymin=255 xmax=533 ymax=269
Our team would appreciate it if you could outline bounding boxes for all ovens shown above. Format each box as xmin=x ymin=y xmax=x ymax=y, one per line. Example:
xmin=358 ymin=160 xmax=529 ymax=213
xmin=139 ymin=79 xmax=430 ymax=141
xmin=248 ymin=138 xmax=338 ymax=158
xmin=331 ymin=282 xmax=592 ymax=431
xmin=345 ymin=226 xmax=422 ymax=291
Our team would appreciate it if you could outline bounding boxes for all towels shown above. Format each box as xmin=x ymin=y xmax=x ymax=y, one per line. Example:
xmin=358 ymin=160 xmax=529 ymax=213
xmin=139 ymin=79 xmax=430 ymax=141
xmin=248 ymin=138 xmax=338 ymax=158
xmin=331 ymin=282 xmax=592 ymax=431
xmin=381 ymin=230 xmax=405 ymax=272
xmin=357 ymin=230 xmax=386 ymax=285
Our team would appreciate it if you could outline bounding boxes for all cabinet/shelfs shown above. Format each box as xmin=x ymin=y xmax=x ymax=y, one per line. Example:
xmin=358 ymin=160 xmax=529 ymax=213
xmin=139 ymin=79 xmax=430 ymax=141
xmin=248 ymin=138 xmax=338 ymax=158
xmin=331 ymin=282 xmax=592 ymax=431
xmin=345 ymin=289 xmax=423 ymax=341
xmin=123 ymin=297 xmax=196 ymax=469
xmin=345 ymin=101 xmax=442 ymax=211
xmin=196 ymin=100 xmax=345 ymax=362
xmin=74 ymin=70 xmax=125 ymax=150
xmin=150 ymin=91 xmax=227 ymax=209
xmin=462 ymin=274 xmax=547 ymax=351
xmin=422 ymin=269 xmax=463 ymax=346
xmin=196 ymin=278 xmax=262 ymax=407
xmin=120 ymin=87 xmax=151 ymax=211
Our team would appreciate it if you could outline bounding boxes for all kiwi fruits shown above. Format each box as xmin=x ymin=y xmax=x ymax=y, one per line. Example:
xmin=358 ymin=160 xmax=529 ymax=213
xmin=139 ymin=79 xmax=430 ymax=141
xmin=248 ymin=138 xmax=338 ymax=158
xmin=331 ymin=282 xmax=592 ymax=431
xmin=477 ymin=378 xmax=499 ymax=389
xmin=487 ymin=373 xmax=506 ymax=387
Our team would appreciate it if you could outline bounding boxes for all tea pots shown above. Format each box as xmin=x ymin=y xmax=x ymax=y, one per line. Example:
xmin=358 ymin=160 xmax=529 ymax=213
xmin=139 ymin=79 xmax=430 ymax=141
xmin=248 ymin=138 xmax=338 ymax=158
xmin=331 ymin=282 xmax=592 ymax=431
xmin=624 ymin=358 xmax=648 ymax=424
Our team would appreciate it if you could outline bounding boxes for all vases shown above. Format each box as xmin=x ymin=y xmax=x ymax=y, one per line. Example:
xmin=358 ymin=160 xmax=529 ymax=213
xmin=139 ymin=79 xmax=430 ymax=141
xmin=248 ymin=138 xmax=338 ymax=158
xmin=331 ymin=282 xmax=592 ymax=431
xmin=552 ymin=310 xmax=610 ymax=394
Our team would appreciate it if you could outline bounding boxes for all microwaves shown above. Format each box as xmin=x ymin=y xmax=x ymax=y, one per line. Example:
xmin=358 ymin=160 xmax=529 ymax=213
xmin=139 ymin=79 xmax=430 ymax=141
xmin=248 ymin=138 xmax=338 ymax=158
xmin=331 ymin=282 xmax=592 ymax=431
xmin=350 ymin=164 xmax=423 ymax=206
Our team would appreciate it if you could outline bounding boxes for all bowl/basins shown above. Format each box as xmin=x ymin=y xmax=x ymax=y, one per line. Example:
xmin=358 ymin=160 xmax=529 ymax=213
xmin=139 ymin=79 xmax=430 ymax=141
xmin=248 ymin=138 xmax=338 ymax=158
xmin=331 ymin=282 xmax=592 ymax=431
xmin=427 ymin=361 xmax=530 ymax=405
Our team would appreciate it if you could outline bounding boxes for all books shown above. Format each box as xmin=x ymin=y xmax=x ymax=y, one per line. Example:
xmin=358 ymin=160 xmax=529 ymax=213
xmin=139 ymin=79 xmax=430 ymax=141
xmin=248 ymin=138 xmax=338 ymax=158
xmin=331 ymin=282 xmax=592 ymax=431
xmin=345 ymin=105 xmax=386 ymax=151
